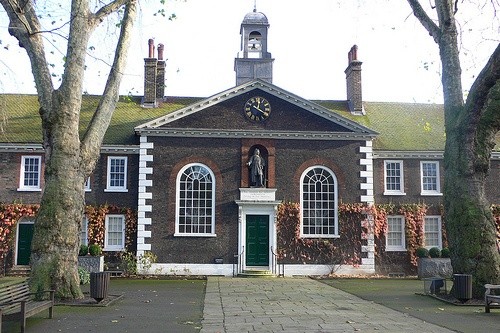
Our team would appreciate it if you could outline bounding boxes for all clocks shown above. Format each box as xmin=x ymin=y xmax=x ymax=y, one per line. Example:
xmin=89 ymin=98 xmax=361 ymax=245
xmin=244 ymin=96 xmax=271 ymax=124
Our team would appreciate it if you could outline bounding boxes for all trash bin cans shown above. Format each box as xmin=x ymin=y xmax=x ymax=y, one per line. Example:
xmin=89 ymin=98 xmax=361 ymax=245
xmin=89 ymin=272 xmax=111 ymax=301
xmin=452 ymin=274 xmax=473 ymax=304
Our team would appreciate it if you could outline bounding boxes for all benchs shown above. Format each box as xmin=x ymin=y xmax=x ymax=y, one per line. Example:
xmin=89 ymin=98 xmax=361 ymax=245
xmin=483 ymin=283 xmax=500 ymax=312
xmin=0 ymin=279 xmax=57 ymax=333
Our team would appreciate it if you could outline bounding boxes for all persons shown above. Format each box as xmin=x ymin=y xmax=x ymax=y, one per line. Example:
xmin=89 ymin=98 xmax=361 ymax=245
xmin=245 ymin=148 xmax=266 ymax=187
